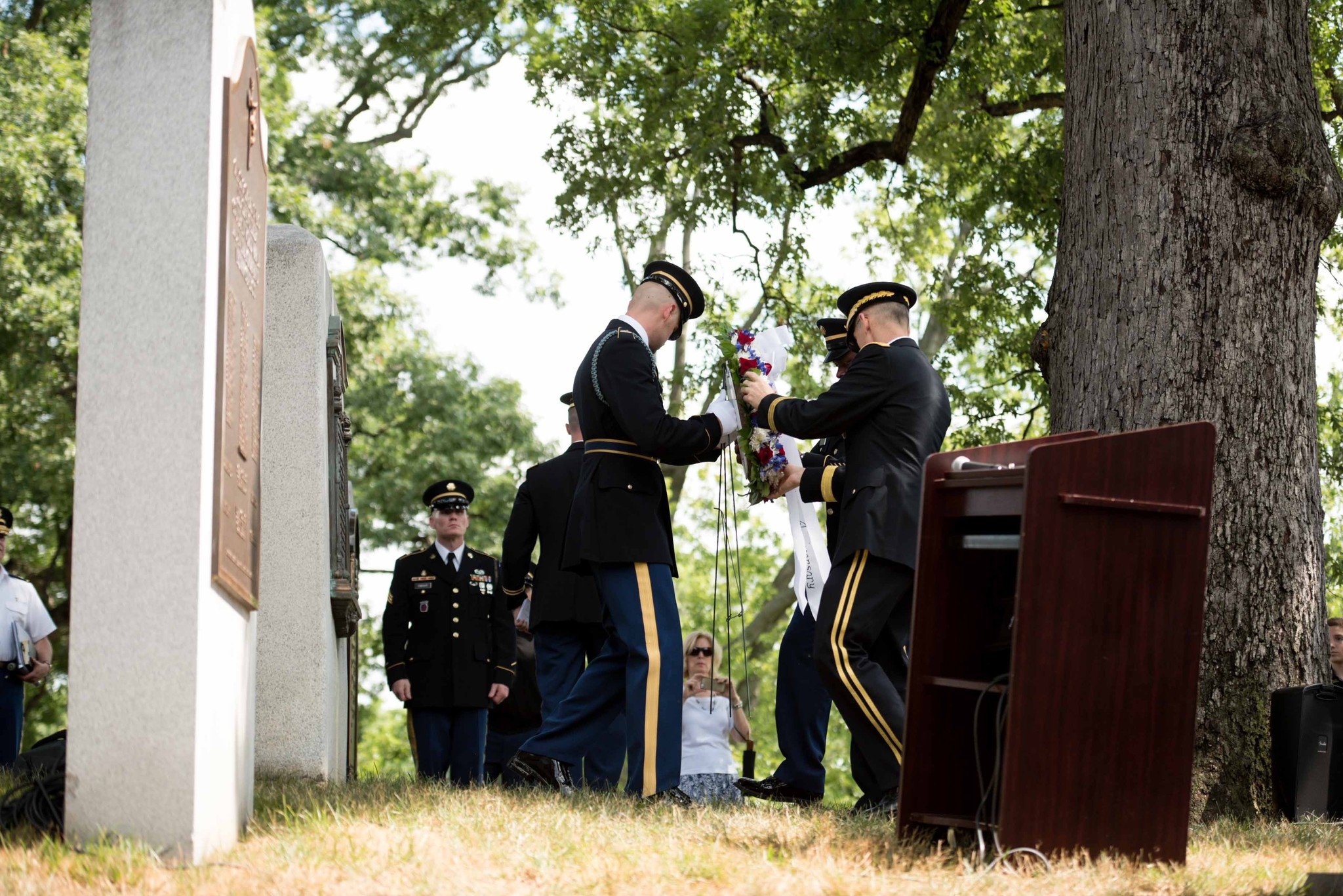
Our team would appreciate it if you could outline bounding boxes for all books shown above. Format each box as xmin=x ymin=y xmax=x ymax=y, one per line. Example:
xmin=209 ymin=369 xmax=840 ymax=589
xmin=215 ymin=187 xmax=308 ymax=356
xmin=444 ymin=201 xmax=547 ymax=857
xmin=12 ymin=621 xmax=41 ymax=687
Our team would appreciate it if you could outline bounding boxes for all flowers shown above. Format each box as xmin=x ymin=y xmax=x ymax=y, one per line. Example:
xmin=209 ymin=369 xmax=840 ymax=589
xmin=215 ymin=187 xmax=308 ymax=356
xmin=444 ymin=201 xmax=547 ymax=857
xmin=713 ymin=319 xmax=788 ymax=506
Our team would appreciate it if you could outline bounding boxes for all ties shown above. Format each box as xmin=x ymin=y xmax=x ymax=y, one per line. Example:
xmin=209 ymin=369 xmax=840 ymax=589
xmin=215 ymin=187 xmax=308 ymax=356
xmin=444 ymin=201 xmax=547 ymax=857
xmin=446 ymin=553 xmax=457 ymax=576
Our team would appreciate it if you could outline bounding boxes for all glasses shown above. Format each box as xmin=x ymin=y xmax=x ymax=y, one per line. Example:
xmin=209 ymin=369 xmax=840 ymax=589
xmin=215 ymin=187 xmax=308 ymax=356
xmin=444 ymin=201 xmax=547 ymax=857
xmin=691 ymin=647 xmax=713 ymax=656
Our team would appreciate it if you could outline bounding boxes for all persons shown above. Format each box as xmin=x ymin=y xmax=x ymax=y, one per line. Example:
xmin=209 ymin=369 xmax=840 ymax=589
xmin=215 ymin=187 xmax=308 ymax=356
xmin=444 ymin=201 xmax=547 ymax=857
xmin=0 ymin=506 xmax=58 ymax=774
xmin=734 ymin=282 xmax=952 ymax=819
xmin=381 ymin=477 xmax=515 ymax=789
xmin=499 ymin=392 xmax=628 ymax=797
xmin=1328 ymin=617 xmax=1343 ymax=690
xmin=679 ymin=630 xmax=750 ymax=807
xmin=504 ymin=262 xmax=738 ymax=803
xmin=483 ymin=558 xmax=536 ymax=783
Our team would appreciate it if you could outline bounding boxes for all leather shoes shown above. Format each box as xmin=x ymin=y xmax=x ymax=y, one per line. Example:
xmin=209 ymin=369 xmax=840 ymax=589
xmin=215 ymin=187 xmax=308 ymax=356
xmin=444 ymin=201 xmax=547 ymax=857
xmin=733 ymin=775 xmax=824 ymax=808
xmin=646 ymin=787 xmax=692 ymax=811
xmin=506 ymin=749 xmax=581 ymax=796
xmin=848 ymin=802 xmax=898 ymax=824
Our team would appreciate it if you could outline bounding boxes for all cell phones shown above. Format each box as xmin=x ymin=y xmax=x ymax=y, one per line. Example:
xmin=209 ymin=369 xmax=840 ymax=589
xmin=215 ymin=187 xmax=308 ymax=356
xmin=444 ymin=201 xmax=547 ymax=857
xmin=700 ymin=676 xmax=725 ymax=693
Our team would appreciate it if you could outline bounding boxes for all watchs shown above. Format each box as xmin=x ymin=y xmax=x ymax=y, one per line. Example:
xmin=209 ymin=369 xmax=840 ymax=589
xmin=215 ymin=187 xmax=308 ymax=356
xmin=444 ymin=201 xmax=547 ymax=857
xmin=42 ymin=660 xmax=53 ymax=668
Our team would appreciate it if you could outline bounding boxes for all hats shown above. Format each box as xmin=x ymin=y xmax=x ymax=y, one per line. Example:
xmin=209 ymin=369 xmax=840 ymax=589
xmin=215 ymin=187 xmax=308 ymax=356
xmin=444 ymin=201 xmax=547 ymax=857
xmin=837 ymin=282 xmax=917 ymax=353
xmin=560 ymin=392 xmax=575 ymax=411
xmin=422 ymin=479 xmax=474 ymax=510
xmin=0 ymin=505 xmax=14 ymax=535
xmin=640 ymin=261 xmax=705 ymax=341
xmin=523 ymin=561 xmax=537 ymax=587
xmin=817 ymin=318 xmax=850 ymax=364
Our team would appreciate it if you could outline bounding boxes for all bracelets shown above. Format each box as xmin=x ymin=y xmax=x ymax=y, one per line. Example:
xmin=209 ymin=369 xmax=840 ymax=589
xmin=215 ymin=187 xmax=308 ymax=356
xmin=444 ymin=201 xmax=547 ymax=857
xmin=732 ymin=702 xmax=743 ymax=709
xmin=695 ymin=693 xmax=716 ymax=711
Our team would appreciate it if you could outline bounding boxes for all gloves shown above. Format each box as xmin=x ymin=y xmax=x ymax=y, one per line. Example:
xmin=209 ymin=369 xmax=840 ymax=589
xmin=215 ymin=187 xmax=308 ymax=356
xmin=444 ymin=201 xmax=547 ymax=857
xmin=716 ymin=428 xmax=737 ymax=450
xmin=706 ymin=389 xmax=738 ymax=437
